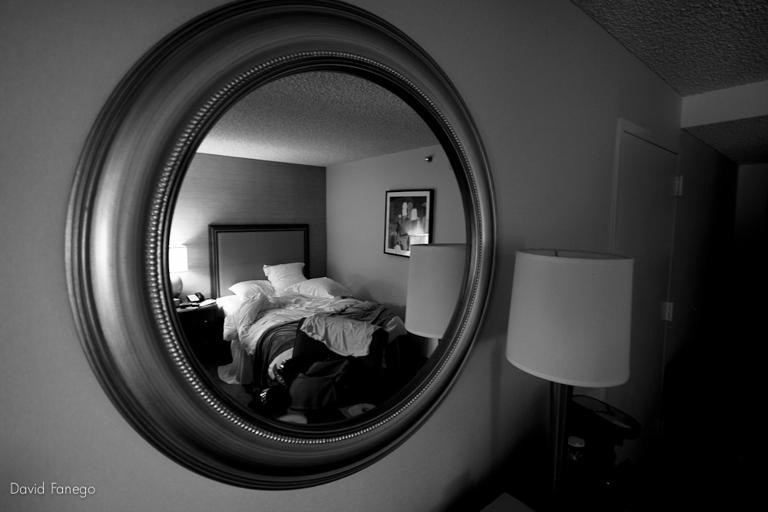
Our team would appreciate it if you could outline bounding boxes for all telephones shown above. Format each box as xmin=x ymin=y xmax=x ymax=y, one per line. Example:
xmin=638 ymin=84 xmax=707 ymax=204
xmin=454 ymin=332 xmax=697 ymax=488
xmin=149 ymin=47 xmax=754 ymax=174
xmin=187 ymin=292 xmax=204 ymax=303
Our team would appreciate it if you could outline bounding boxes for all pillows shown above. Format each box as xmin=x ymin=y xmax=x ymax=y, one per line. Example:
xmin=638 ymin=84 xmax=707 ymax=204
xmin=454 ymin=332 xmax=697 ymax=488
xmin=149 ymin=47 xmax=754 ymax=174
xmin=230 ymin=276 xmax=270 ymax=303
xmin=293 ymin=276 xmax=346 ymax=302
xmin=260 ymin=264 xmax=302 ymax=295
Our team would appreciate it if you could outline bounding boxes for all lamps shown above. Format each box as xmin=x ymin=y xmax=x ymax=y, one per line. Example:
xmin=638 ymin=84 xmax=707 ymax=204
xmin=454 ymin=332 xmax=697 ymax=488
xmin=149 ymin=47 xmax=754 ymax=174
xmin=505 ymin=248 xmax=632 ymax=501
xmin=167 ymin=241 xmax=186 ymax=293
xmin=403 ymin=246 xmax=465 ymax=340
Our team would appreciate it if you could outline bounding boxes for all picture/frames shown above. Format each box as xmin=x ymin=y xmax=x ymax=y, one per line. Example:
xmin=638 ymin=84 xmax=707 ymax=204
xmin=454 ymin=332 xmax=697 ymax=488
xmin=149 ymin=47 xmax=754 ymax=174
xmin=381 ymin=187 xmax=434 ymax=257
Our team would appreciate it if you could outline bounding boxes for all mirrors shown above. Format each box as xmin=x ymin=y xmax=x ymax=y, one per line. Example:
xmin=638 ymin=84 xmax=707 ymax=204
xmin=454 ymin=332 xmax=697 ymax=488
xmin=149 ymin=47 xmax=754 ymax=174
xmin=65 ymin=1 xmax=496 ymax=490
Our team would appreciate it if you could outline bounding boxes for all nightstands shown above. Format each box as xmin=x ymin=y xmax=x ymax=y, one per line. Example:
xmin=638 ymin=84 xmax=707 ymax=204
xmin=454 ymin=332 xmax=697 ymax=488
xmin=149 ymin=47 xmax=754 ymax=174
xmin=175 ymin=299 xmax=219 ymax=378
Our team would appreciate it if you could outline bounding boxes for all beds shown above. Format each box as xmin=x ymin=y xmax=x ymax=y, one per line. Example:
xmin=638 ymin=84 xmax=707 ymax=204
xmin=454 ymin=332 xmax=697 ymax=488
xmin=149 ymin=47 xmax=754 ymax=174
xmin=208 ymin=222 xmax=406 ymax=392
